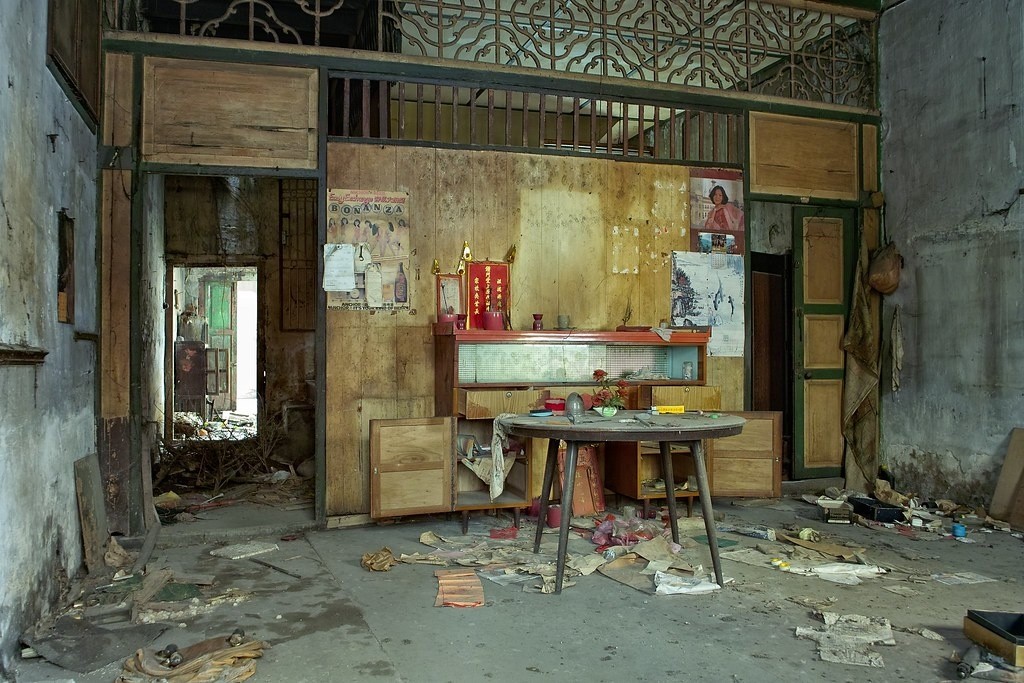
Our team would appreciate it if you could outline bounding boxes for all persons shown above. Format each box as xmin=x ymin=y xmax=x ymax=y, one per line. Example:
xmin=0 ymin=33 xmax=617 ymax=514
xmin=705 ymin=186 xmax=744 ymax=230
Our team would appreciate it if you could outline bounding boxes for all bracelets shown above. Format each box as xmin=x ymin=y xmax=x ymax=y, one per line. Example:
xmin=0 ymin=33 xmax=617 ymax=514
xmin=713 ymin=208 xmax=716 ymax=210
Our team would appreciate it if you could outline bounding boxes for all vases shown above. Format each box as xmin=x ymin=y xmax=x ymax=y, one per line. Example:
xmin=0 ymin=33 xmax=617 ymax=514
xmin=592 ymin=406 xmax=618 ymax=418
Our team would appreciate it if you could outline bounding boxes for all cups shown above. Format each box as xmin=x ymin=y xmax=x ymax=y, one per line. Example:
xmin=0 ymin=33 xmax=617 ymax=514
xmin=682 ymin=361 xmax=692 ymax=380
xmin=483 ymin=312 xmax=505 ymax=329
xmin=952 ymin=524 xmax=966 ymax=537
xmin=439 ymin=314 xmax=458 ymax=331
xmin=557 ymin=314 xmax=570 ymax=328
xmin=687 ymin=475 xmax=699 ymax=491
xmin=921 ymin=501 xmax=937 ymax=513
xmin=547 ymin=504 xmax=563 ymax=528
xmin=623 ymin=507 xmax=636 ymax=522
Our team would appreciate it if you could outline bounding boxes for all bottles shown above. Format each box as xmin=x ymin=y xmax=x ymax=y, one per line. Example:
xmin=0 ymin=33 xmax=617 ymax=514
xmin=658 ymin=316 xmax=668 ymax=329
xmin=636 ymin=509 xmax=657 ymax=519
xmin=961 ymin=514 xmax=978 ymax=519
xmin=532 ymin=313 xmax=543 ymax=331
xmin=393 ymin=262 xmax=407 ymax=302
xmin=456 ymin=314 xmax=468 ymax=330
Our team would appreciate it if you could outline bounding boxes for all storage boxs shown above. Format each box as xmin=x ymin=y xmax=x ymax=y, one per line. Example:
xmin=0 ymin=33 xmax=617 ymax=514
xmin=818 ymin=499 xmax=854 ymax=525
xmin=966 ymin=609 xmax=1024 ymax=668
xmin=848 ymin=497 xmax=908 ymax=523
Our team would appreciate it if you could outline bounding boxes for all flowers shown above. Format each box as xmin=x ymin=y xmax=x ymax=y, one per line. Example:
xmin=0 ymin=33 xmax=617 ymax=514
xmin=591 ymin=370 xmax=630 ymax=415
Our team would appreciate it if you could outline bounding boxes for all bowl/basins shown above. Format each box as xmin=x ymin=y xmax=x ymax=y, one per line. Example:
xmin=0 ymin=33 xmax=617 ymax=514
xmin=544 ymin=398 xmax=566 ymax=411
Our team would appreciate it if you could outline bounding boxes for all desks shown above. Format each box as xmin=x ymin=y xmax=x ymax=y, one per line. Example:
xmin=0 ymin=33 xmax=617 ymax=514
xmin=499 ymin=412 xmax=747 ymax=595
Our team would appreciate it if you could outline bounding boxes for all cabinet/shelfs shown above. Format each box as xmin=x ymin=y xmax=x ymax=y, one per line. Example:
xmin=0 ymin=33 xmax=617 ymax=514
xmin=174 ymin=341 xmax=230 ymax=422
xmin=368 ymin=324 xmax=783 ymax=532
